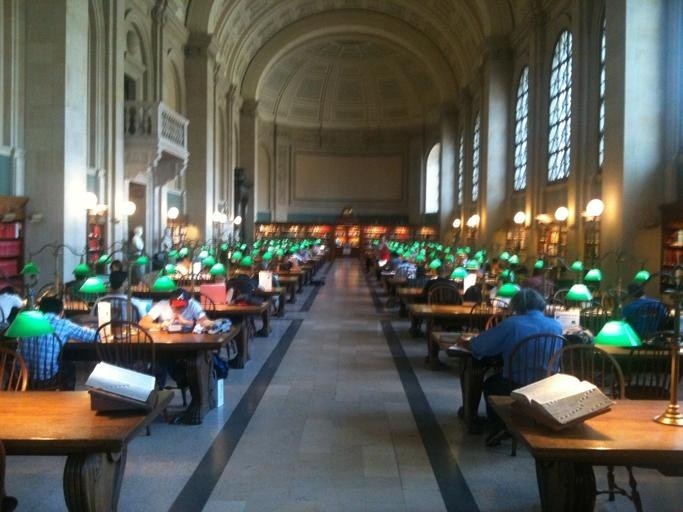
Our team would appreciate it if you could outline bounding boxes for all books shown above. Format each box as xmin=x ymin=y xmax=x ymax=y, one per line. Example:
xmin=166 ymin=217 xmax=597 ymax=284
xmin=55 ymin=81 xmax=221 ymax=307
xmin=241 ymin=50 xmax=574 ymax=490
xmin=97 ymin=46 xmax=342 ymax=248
xmin=0 ymin=221 xmax=24 ymax=293
xmin=82 ymin=359 xmax=157 ymax=401
xmin=661 ymin=224 xmax=683 ymax=294
xmin=509 ymin=372 xmax=614 ymax=431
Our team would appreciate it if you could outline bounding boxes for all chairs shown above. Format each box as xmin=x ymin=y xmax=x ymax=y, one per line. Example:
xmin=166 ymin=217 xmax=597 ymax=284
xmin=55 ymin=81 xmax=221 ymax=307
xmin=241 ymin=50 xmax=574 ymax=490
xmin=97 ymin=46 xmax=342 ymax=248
xmin=0 ymin=320 xmax=174 ymax=435
xmin=546 ymin=344 xmax=643 ymax=512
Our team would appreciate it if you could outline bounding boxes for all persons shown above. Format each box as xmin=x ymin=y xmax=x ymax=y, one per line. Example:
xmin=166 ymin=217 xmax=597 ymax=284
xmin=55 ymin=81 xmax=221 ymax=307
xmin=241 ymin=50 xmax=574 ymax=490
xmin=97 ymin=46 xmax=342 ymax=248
xmin=613 ymin=284 xmax=668 ymax=383
xmin=456 ymin=288 xmax=563 ymax=433
xmin=160 ymin=227 xmax=171 ymax=247
xmin=365 ymin=232 xmax=555 ymax=335
xmin=14 ymin=296 xmax=100 ymax=391
xmin=88 ymin=245 xmax=322 ymax=392
xmin=0 ymin=285 xmax=24 ymax=325
xmin=131 ymin=226 xmax=144 ymax=250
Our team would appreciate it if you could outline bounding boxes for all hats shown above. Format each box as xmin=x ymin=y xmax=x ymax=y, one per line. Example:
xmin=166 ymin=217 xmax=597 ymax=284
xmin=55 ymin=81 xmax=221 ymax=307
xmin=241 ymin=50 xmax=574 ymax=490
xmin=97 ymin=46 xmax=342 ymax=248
xmin=169 ymin=289 xmax=190 ymax=307
xmin=511 ymin=288 xmax=546 ymax=309
xmin=627 ymin=284 xmax=643 ymax=296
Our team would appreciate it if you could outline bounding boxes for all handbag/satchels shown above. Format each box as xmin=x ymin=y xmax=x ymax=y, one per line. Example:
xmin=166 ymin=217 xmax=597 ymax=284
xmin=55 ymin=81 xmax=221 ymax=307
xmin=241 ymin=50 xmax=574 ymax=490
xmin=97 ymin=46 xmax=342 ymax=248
xmin=209 ymin=354 xmax=229 ymax=408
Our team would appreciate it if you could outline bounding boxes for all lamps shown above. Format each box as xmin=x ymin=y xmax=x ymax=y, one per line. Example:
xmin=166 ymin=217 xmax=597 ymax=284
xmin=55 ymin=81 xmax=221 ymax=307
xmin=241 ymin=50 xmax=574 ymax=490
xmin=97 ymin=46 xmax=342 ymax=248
xmin=565 ymin=283 xmax=594 ymax=302
xmin=85 ymin=192 xmax=98 ymax=208
xmin=555 ymin=206 xmax=569 ymax=220
xmin=466 ymin=214 xmax=480 ymax=228
xmin=453 ymin=218 xmax=461 ymax=229
xmin=73 ymin=264 xmax=92 ymax=276
xmin=586 ymin=199 xmax=605 ymax=217
xmin=221 ymin=238 xmax=323 ymax=268
xmin=571 ymin=261 xmax=584 ymax=271
xmin=534 ymin=260 xmax=544 ymax=269
xmin=374 ymin=239 xmax=379 ymax=245
xmin=500 ymin=251 xmax=520 ymax=265
xmin=496 ymin=284 xmax=520 ymax=297
xmin=124 ymin=201 xmax=137 ymax=217
xmin=386 ymin=240 xmax=455 ymax=269
xmin=592 ymin=320 xmax=641 ymax=344
xmin=167 ymin=206 xmax=180 ymax=220
xmin=234 ymin=215 xmax=242 ymax=226
xmin=584 ymin=269 xmax=604 ymax=281
xmin=136 ymin=256 xmax=149 ymax=267
xmin=99 ymin=255 xmax=111 ymax=266
xmin=450 ymin=246 xmax=486 ymax=279
xmin=198 ymin=246 xmax=225 ymax=275
xmin=20 ymin=262 xmax=39 ymax=275
xmin=152 ymin=249 xmax=180 ymax=293
xmin=635 ymin=271 xmax=651 ymax=281
xmin=78 ymin=276 xmax=106 ymax=293
xmin=500 ymin=269 xmax=515 ymax=281
xmin=6 ymin=311 xmax=54 ymax=338
xmin=514 ymin=212 xmax=526 ymax=224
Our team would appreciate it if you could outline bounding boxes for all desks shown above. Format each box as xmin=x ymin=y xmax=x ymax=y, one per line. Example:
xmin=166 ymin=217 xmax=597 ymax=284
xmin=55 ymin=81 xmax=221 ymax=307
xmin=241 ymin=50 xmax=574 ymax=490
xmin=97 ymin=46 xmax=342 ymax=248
xmin=0 ymin=391 xmax=176 ymax=512
xmin=488 ymin=392 xmax=683 ymax=512
